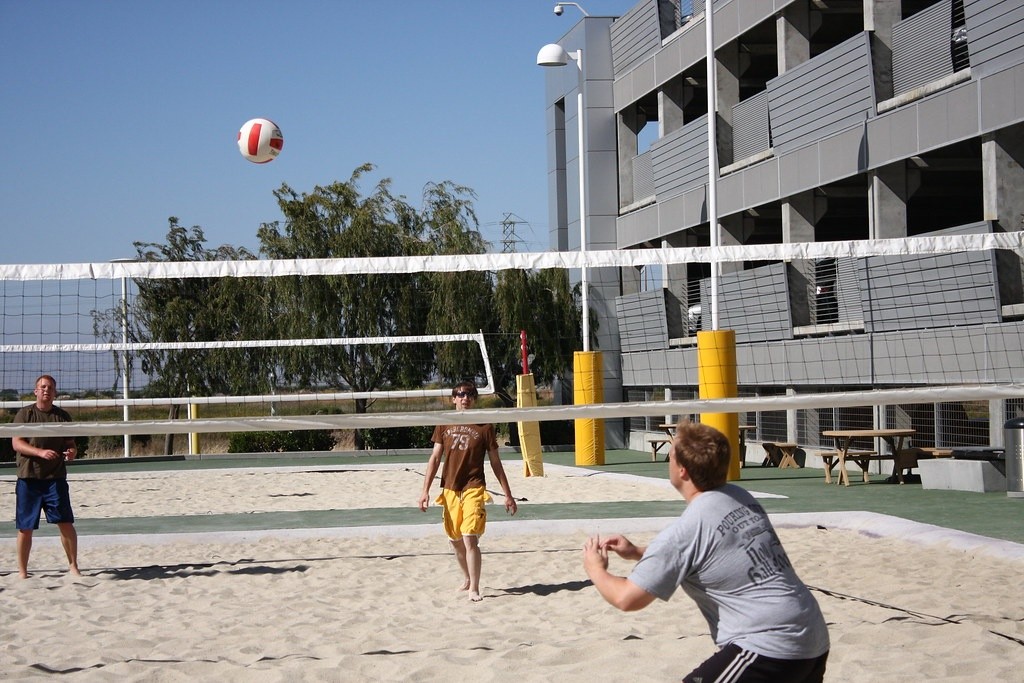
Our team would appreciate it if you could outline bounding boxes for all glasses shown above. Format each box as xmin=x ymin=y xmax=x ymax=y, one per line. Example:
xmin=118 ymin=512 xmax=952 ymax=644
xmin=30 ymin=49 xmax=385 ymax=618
xmin=456 ymin=391 xmax=476 ymax=398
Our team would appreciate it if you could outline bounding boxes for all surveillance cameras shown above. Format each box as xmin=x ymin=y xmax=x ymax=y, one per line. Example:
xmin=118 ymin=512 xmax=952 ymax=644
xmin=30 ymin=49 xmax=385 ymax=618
xmin=554 ymin=6 xmax=564 ymax=16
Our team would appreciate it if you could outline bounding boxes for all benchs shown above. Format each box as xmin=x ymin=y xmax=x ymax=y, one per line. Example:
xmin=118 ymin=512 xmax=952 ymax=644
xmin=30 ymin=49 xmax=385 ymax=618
xmin=647 ymin=440 xmax=670 ymax=462
xmin=761 ymin=442 xmax=801 ymax=469
xmin=813 ymin=447 xmax=953 ymax=484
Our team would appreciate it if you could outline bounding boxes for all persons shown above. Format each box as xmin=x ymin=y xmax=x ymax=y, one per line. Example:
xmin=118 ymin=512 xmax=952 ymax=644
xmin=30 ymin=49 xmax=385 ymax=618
xmin=419 ymin=381 xmax=518 ymax=601
xmin=12 ymin=375 xmax=85 ymax=579
xmin=583 ymin=421 xmax=830 ymax=683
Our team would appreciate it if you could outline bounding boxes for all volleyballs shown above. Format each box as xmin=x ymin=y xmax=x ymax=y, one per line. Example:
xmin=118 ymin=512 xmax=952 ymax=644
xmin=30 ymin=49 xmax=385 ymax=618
xmin=235 ymin=118 xmax=285 ymax=167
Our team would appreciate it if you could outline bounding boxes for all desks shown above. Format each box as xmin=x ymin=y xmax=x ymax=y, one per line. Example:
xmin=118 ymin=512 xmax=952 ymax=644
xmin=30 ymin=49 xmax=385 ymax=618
xmin=822 ymin=428 xmax=916 ymax=488
xmin=659 ymin=424 xmax=757 ymax=468
